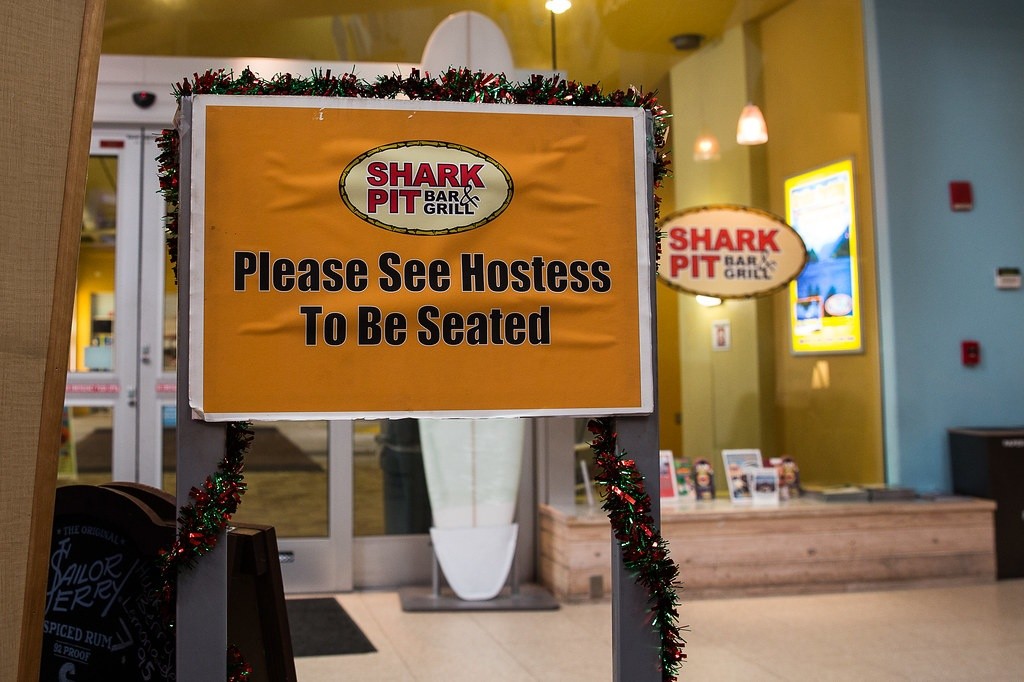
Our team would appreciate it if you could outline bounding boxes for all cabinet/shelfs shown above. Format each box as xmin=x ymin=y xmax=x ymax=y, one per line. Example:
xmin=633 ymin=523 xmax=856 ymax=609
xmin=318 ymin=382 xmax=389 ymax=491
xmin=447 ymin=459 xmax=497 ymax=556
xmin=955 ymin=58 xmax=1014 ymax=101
xmin=947 ymin=426 xmax=1024 ymax=580
xmin=538 ymin=496 xmax=997 ymax=606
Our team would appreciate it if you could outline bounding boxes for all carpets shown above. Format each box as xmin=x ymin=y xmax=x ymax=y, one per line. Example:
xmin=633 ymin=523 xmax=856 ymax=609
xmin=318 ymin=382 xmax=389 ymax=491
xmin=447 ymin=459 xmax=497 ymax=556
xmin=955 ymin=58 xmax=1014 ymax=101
xmin=75 ymin=425 xmax=326 ymax=474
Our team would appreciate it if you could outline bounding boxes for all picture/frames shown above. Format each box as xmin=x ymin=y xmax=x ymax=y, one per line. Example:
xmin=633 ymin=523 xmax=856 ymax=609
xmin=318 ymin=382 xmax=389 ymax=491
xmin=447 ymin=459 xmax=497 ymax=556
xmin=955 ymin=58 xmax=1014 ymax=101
xmin=784 ymin=153 xmax=866 ymax=357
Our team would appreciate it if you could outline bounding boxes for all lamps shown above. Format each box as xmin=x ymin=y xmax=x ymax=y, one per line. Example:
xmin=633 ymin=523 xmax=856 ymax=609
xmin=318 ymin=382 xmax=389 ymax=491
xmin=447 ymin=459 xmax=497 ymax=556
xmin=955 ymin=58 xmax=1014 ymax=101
xmin=693 ymin=38 xmax=724 ymax=164
xmin=736 ymin=103 xmax=769 ymax=146
